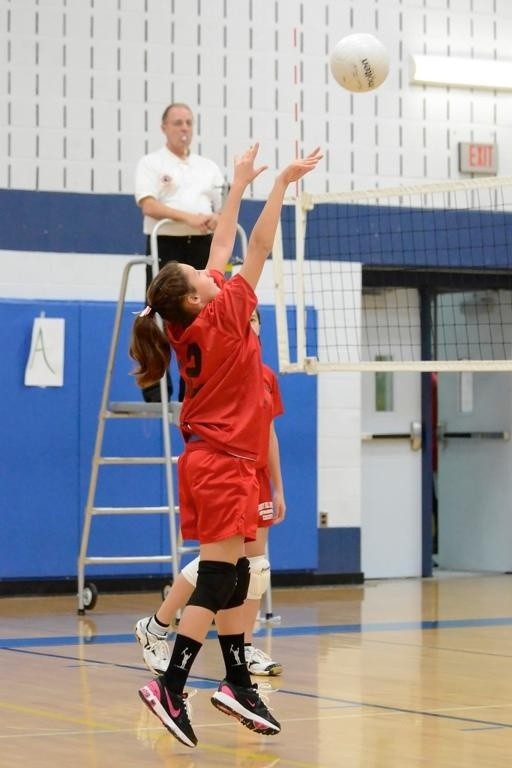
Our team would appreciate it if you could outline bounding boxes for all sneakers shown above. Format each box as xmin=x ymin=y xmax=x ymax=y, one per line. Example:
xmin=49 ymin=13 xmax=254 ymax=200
xmin=149 ymin=388 xmax=172 ymax=403
xmin=134 ymin=615 xmax=172 ymax=676
xmin=242 ymin=645 xmax=284 ymax=677
xmin=211 ymin=678 xmax=282 ymax=737
xmin=137 ymin=672 xmax=199 ymax=749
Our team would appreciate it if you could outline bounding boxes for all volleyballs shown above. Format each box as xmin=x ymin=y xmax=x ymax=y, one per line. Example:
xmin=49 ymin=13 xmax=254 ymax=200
xmin=330 ymin=33 xmax=389 ymax=93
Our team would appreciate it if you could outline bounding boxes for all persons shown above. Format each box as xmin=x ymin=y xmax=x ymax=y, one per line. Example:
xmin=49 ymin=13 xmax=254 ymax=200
xmin=134 ymin=104 xmax=227 ymax=407
xmin=134 ymin=301 xmax=283 ymax=677
xmin=133 ymin=145 xmax=324 ymax=749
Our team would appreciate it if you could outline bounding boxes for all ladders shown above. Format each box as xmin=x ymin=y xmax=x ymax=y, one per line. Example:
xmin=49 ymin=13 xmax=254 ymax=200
xmin=76 ymin=218 xmax=274 ymax=627
